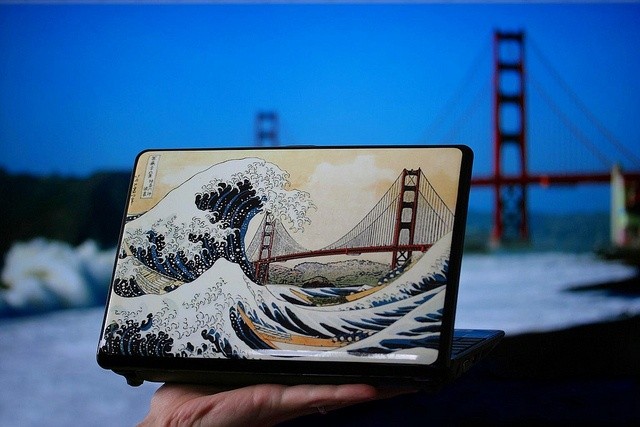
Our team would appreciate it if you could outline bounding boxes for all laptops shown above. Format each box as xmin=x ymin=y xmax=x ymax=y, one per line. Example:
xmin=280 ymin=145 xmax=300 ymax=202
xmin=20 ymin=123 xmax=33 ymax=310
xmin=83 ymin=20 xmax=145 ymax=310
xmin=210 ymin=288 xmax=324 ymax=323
xmin=96 ymin=144 xmax=504 ymax=395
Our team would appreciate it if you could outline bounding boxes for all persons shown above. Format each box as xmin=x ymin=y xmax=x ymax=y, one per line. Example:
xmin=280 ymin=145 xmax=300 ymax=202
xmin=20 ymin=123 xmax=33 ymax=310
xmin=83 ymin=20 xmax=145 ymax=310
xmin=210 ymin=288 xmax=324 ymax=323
xmin=133 ymin=380 xmax=419 ymax=426
